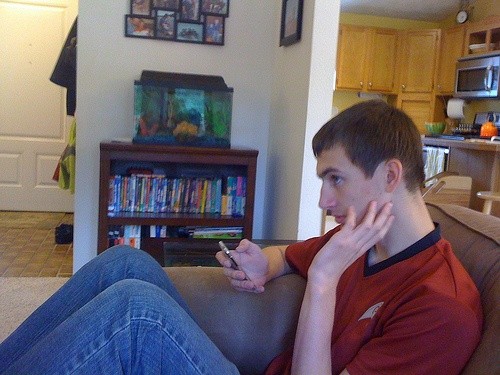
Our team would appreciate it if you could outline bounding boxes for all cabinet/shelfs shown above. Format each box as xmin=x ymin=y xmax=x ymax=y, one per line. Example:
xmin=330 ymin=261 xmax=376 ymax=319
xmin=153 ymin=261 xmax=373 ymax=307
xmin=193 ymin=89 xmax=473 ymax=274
xmin=396 ymin=92 xmax=435 ymax=134
xmin=335 ymin=24 xmax=400 ymax=94
xmin=96 ymin=138 xmax=259 ymax=268
xmin=402 ymin=30 xmax=441 ymax=95
xmin=441 ymin=26 xmax=464 ymax=94
xmin=463 ymin=23 xmax=500 ymax=55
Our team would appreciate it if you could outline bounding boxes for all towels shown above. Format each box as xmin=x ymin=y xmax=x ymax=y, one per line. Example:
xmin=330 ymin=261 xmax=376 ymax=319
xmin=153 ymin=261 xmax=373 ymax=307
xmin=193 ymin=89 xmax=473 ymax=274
xmin=424 ymin=147 xmax=446 ymax=186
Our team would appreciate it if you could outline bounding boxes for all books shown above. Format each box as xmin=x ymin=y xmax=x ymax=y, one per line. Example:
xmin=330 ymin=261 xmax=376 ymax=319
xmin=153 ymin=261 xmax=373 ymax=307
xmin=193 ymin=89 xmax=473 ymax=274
xmin=107 ymin=226 xmax=243 ymax=249
xmin=109 ymin=169 xmax=247 ymax=217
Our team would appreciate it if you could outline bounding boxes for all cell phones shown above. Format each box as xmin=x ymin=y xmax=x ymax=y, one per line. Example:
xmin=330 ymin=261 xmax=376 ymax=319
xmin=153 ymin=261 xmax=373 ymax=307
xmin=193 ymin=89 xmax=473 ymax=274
xmin=218 ymin=241 xmax=258 ymax=289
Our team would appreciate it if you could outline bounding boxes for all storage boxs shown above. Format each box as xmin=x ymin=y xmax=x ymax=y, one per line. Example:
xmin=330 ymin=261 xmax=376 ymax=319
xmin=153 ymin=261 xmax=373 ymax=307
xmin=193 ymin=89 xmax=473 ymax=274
xmin=132 ymin=70 xmax=235 ymax=149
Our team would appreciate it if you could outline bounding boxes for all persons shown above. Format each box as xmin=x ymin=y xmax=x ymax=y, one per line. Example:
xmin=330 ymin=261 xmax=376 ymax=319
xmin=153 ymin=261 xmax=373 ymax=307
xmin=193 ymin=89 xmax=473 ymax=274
xmin=1 ymin=98 xmax=483 ymax=375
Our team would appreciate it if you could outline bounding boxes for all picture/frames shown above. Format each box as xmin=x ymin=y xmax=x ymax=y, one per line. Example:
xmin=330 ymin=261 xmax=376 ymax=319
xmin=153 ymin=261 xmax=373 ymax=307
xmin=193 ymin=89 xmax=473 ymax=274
xmin=125 ymin=0 xmax=229 ymax=46
xmin=278 ymin=0 xmax=303 ymax=47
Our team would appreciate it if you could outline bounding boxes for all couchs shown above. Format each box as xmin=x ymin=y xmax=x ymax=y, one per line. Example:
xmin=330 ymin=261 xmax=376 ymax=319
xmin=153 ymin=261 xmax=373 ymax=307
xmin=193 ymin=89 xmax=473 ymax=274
xmin=162 ymin=200 xmax=500 ymax=375
